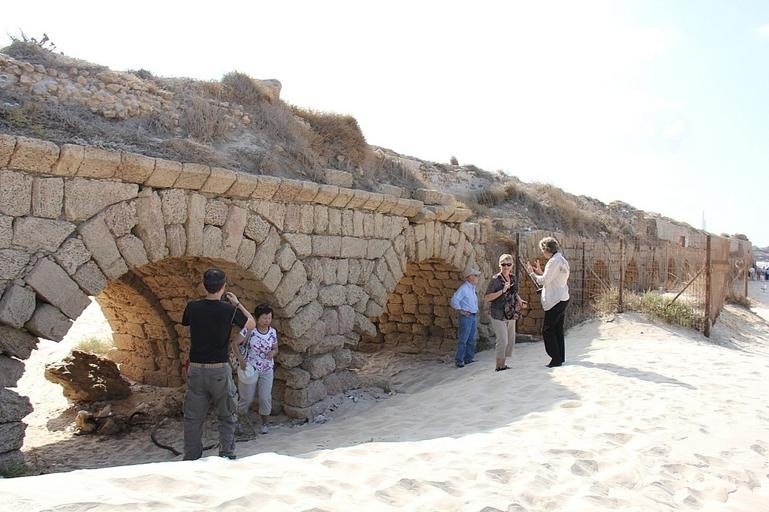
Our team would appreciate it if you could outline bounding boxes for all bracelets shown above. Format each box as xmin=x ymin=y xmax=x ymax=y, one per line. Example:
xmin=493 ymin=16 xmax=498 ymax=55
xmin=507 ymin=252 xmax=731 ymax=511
xmin=235 ymin=303 xmax=240 ymax=308
xmin=502 ymin=290 xmax=504 ymax=294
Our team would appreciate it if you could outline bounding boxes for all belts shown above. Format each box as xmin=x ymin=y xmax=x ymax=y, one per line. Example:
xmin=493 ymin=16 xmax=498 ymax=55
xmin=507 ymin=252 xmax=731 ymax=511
xmin=189 ymin=362 xmax=229 ymax=368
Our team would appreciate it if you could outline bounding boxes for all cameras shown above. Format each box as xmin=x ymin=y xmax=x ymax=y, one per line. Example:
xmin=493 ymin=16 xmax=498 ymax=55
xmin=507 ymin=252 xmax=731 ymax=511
xmin=220 ymin=293 xmax=230 ymax=302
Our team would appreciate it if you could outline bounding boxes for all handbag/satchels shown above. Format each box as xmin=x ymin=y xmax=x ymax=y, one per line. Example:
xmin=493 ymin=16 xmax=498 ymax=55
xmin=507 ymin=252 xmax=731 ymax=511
xmin=504 ymin=302 xmax=522 ymax=321
xmin=229 ymin=346 xmax=247 ymax=369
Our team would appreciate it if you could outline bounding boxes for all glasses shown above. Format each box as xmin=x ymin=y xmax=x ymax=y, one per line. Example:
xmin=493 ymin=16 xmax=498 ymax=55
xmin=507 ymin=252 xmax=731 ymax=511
xmin=501 ymin=263 xmax=512 ymax=266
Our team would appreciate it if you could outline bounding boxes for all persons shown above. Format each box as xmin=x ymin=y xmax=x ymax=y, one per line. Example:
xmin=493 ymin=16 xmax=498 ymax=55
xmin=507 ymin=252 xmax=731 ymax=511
xmin=748 ymin=261 xmax=769 ymax=282
xmin=230 ymin=302 xmax=279 ymax=435
xmin=524 ymin=237 xmax=570 ymax=368
xmin=451 ymin=268 xmax=482 ymax=367
xmin=484 ymin=254 xmax=528 ymax=372
xmin=182 ymin=268 xmax=256 ymax=461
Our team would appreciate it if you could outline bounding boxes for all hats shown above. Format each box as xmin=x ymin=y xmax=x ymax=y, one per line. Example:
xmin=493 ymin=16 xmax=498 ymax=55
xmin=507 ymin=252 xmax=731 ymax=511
xmin=463 ymin=268 xmax=481 ymax=278
xmin=237 ymin=362 xmax=259 ymax=385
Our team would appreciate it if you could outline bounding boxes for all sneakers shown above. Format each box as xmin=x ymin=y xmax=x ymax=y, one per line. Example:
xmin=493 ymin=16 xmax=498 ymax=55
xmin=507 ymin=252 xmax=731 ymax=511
xmin=259 ymin=423 xmax=268 ymax=434
